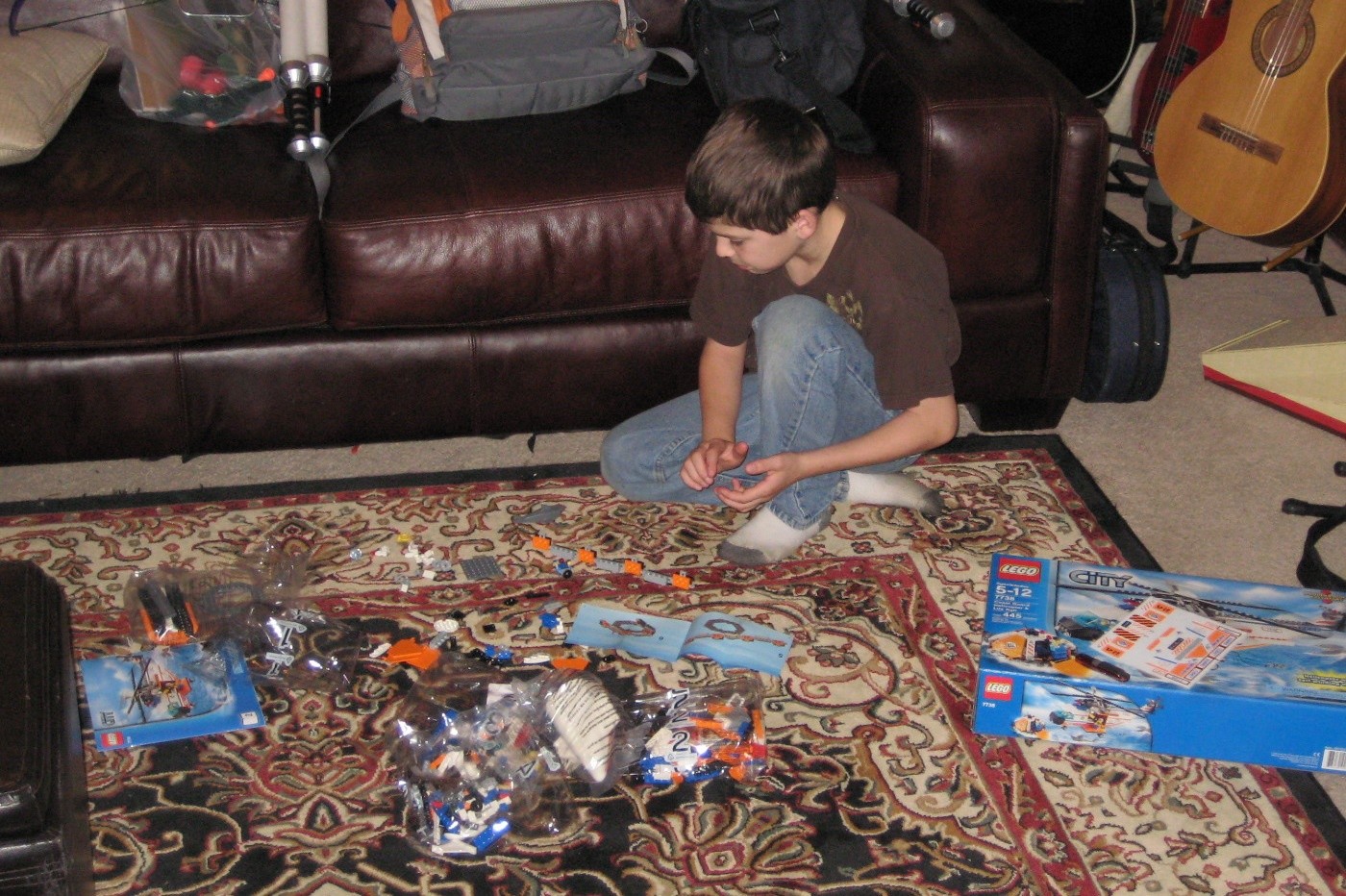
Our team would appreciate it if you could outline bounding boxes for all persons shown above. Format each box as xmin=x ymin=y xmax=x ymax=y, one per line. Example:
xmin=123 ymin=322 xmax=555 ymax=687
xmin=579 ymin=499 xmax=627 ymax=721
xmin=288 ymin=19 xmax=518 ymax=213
xmin=599 ymin=98 xmax=964 ymax=568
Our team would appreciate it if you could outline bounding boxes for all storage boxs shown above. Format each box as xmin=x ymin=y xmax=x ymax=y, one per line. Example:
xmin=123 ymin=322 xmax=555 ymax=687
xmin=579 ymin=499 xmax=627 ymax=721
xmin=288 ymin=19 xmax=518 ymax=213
xmin=972 ymin=554 xmax=1346 ymax=774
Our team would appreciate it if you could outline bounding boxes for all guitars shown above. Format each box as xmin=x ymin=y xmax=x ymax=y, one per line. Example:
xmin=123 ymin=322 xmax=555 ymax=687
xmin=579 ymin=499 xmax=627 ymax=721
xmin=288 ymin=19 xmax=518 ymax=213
xmin=1129 ymin=1 xmax=1346 ymax=246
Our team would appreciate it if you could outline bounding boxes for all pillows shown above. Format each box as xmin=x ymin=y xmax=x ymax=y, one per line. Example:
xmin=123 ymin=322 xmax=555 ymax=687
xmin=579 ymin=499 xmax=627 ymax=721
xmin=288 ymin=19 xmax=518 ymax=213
xmin=0 ymin=24 xmax=115 ymax=167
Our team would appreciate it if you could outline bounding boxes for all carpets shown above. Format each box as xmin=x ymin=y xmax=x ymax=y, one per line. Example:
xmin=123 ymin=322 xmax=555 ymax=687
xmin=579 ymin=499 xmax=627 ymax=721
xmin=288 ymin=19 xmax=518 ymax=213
xmin=0 ymin=434 xmax=1346 ymax=896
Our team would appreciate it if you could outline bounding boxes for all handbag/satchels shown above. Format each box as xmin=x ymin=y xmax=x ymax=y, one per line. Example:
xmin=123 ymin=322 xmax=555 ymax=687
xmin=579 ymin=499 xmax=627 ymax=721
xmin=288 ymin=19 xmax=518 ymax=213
xmin=1070 ymin=230 xmax=1170 ymax=402
xmin=687 ymin=0 xmax=864 ymax=136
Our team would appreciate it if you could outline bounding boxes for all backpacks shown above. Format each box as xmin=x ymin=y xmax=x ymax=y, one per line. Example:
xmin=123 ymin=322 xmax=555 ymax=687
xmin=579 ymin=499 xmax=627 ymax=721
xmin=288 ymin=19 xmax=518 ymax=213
xmin=394 ymin=0 xmax=697 ymax=122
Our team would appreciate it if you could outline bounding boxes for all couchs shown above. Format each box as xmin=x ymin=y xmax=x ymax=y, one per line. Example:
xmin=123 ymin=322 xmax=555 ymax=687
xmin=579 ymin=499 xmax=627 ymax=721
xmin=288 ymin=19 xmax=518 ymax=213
xmin=0 ymin=0 xmax=1109 ymax=468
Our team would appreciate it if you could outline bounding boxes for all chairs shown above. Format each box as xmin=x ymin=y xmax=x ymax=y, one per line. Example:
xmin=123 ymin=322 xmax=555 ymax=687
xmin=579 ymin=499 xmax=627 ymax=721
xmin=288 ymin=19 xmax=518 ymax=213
xmin=0 ymin=559 xmax=94 ymax=896
